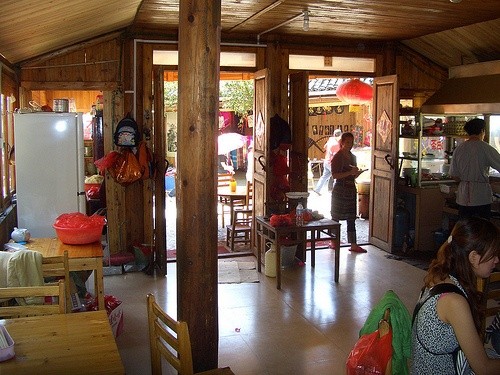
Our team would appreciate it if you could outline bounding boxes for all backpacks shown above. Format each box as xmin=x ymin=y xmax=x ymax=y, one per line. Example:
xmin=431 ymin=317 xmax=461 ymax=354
xmin=114 ymin=112 xmax=139 ymax=146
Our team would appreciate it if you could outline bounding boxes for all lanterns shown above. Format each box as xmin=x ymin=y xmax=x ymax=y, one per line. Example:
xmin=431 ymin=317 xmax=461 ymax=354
xmin=337 ymin=78 xmax=374 ymax=113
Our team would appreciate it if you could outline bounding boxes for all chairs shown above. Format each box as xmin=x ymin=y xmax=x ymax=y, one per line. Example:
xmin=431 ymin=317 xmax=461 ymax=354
xmin=218 ymin=174 xmax=253 ymax=251
xmin=146 ymin=293 xmax=236 ymax=375
xmin=0 ymin=249 xmax=72 ymax=320
xmin=480 ymin=272 xmax=500 ymax=336
xmin=358 ymin=290 xmax=413 ymax=375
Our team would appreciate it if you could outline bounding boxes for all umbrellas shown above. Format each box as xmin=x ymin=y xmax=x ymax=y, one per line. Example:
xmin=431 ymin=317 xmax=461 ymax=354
xmin=218 ymin=132 xmax=248 ymax=161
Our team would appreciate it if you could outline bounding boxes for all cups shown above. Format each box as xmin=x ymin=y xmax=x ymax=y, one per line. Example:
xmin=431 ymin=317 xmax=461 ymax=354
xmin=231 ymin=182 xmax=237 ymax=191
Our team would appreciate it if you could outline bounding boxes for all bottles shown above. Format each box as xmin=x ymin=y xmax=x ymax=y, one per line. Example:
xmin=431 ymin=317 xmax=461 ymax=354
xmin=265 ymin=244 xmax=277 ymax=277
xmin=296 ymin=201 xmax=304 ymax=227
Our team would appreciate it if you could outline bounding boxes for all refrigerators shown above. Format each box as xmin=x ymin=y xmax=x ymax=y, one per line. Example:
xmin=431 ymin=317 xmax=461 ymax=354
xmin=12 ymin=111 xmax=86 ymax=238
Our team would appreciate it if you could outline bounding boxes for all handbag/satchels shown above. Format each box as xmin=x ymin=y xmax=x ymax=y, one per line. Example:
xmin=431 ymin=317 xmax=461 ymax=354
xmin=346 ymin=325 xmax=392 ymax=375
xmin=94 ymin=149 xmax=144 ymax=187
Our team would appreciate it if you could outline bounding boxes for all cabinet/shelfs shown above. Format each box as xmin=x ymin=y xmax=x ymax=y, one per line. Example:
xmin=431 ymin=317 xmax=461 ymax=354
xmin=395 ymin=108 xmax=474 ymax=253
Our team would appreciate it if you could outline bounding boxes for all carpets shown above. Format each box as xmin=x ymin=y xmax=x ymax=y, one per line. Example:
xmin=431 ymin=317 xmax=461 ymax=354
xmin=384 ymin=254 xmax=500 ymax=305
xmin=306 ymin=241 xmax=345 ymax=248
xmin=167 ymin=245 xmax=229 ymax=258
xmin=218 ymin=261 xmax=260 ymax=283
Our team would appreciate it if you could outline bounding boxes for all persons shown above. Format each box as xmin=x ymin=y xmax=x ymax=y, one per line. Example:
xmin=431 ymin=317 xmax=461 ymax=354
xmin=246 ymin=142 xmax=253 ymax=185
xmin=448 ymin=119 xmax=500 ymax=225
xmin=409 ymin=215 xmax=500 ymax=375
xmin=313 ymin=130 xmax=367 ymax=253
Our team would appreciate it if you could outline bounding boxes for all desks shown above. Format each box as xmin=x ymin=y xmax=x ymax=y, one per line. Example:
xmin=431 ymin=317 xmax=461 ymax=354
xmin=311 ymin=160 xmax=324 ymax=178
xmin=256 ymin=214 xmax=341 ymax=290
xmin=0 ymin=310 xmax=129 ymax=375
xmin=5 ymin=239 xmax=106 ymax=311
xmin=217 ymin=185 xmax=252 ymax=225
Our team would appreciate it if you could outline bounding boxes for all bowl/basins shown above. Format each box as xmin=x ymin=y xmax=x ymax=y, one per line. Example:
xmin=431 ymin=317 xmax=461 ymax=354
xmin=53 ymin=219 xmax=105 ymax=245
xmin=84 ymin=183 xmax=101 ymax=199
xmin=402 ymin=167 xmax=416 ymax=176
xmin=432 ymin=173 xmax=443 ymax=178
xmin=421 ymin=169 xmax=430 ymax=174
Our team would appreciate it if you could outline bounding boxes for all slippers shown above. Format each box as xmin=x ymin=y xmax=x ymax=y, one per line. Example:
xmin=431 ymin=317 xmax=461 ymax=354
xmin=349 ymin=246 xmax=366 ymax=252
